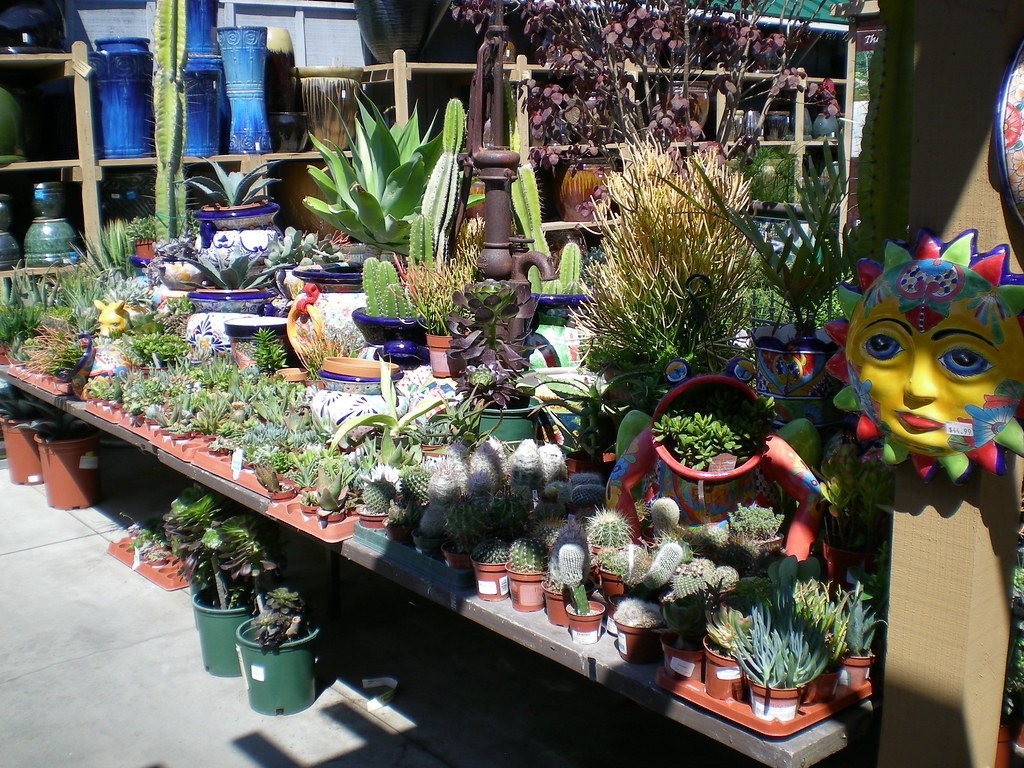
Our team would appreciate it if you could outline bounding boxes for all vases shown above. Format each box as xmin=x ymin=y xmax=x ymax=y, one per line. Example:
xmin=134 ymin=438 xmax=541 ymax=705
xmin=312 ymin=356 xmax=409 ymax=451
xmin=102 ymin=164 xmax=338 ymax=237
xmin=0 ymin=181 xmax=79 ymax=272
xmin=224 ymin=316 xmax=286 ymax=371
xmin=94 ymin=1 xmax=364 ymax=158
xmin=553 ymin=39 xmax=839 ymax=222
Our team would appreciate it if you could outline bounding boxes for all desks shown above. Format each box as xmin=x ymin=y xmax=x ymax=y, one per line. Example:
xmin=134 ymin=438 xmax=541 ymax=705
xmin=1 ymin=365 xmax=879 ymax=768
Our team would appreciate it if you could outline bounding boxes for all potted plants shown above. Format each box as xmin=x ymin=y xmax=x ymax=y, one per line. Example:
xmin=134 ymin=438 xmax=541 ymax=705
xmin=0 ymin=0 xmax=1024 ymax=768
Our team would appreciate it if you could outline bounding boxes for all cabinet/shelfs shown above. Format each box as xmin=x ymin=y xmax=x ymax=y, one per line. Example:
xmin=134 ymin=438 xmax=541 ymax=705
xmin=0 ymin=41 xmax=857 ymax=277
